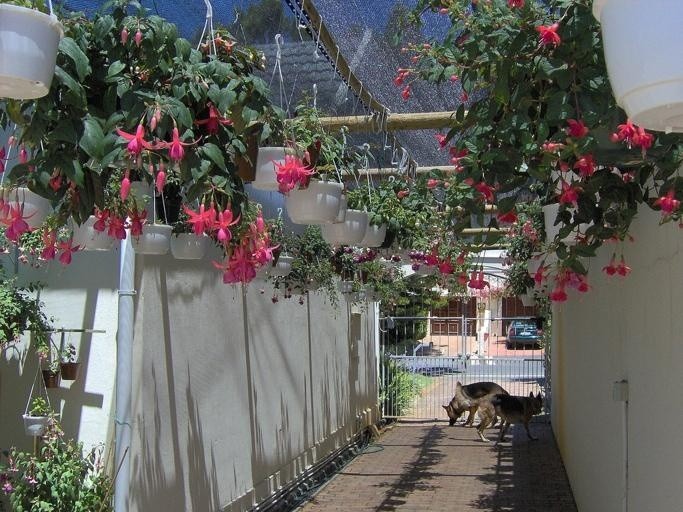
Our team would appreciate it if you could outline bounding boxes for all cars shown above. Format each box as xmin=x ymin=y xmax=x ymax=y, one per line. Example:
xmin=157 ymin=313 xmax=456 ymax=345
xmin=505 ymin=320 xmax=545 ymax=350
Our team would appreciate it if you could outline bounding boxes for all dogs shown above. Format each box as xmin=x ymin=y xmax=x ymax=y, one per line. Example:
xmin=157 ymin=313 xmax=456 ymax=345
xmin=453 ymin=382 xmax=543 ymax=450
xmin=441 ymin=381 xmax=510 ymax=429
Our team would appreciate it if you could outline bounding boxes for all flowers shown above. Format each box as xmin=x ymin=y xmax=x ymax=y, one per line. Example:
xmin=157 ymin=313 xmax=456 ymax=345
xmin=0 ymin=0 xmax=682 ymax=307
xmin=0 ymin=409 xmax=115 ymax=512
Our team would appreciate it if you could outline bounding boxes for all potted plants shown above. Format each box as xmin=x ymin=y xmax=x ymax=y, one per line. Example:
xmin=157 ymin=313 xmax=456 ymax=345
xmin=41 ymin=343 xmax=79 ymax=388
xmin=21 ymin=397 xmax=51 ymax=436
xmin=0 ymin=261 xmax=54 ymax=348
